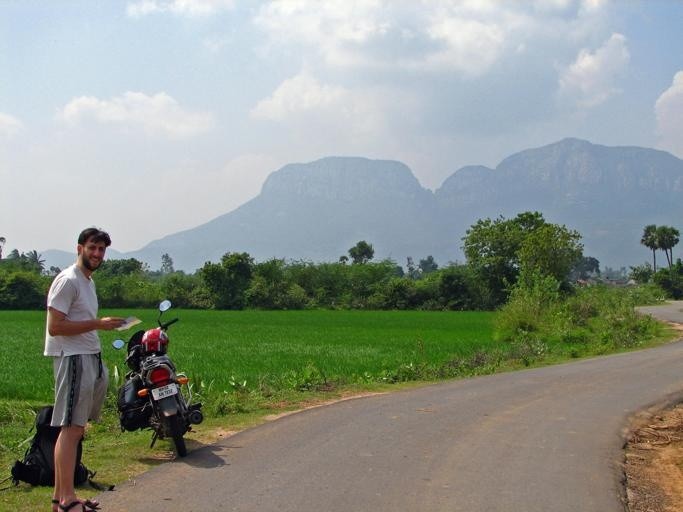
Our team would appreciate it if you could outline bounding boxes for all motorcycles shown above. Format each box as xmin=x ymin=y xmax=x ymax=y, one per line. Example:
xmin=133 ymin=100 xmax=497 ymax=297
xmin=111 ymin=300 xmax=203 ymax=458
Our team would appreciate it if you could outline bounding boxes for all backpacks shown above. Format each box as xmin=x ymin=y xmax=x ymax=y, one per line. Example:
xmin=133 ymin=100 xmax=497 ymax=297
xmin=11 ymin=405 xmax=89 ymax=491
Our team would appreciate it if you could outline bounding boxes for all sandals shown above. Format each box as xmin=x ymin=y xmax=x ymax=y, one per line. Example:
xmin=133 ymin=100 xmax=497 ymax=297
xmin=52 ymin=499 xmax=99 ymax=512
xmin=58 ymin=502 xmax=97 ymax=512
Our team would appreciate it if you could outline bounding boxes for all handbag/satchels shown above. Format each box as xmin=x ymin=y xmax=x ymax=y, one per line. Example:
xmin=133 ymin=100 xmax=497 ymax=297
xmin=117 ymin=375 xmax=154 ymax=433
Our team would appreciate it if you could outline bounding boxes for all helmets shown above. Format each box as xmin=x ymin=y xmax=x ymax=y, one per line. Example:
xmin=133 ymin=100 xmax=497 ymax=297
xmin=140 ymin=329 xmax=169 ymax=353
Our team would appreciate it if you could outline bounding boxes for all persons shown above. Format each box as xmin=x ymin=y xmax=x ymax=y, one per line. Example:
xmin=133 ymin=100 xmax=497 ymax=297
xmin=43 ymin=228 xmax=126 ymax=511
